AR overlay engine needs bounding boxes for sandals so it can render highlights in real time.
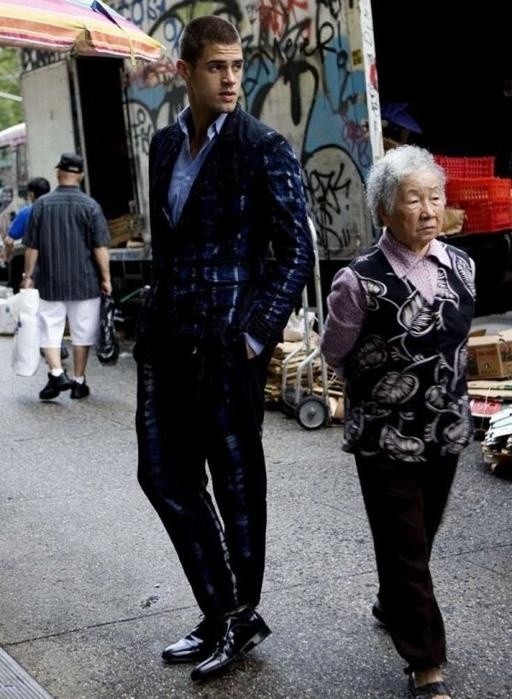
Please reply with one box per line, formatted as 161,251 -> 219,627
405,654 -> 454,699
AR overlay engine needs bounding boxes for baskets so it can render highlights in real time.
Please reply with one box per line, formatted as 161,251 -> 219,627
459,206 -> 512,232
444,178 -> 512,205
430,153 -> 495,178
381,100 -> 421,158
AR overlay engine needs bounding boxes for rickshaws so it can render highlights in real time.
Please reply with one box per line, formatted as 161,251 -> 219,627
278,216 -> 344,431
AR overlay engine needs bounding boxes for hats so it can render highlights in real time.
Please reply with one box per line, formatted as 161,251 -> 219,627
54,153 -> 86,174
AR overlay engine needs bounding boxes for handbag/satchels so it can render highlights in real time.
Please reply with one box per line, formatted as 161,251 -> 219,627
98,290 -> 119,366
13,284 -> 41,377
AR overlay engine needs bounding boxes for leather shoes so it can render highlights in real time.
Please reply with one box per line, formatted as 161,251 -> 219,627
157,612 -> 218,660
194,608 -> 270,683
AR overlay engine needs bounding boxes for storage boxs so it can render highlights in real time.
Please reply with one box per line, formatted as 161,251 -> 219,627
464,329 -> 512,378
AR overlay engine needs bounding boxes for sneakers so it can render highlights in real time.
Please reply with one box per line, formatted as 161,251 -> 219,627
70,373 -> 91,399
38,370 -> 70,400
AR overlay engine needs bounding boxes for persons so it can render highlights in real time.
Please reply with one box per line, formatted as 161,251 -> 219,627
3,153 -> 113,399
132,16 -> 316,681
321,144 -> 479,699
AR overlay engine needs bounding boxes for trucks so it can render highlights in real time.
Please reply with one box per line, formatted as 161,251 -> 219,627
0,0 -> 512,267
